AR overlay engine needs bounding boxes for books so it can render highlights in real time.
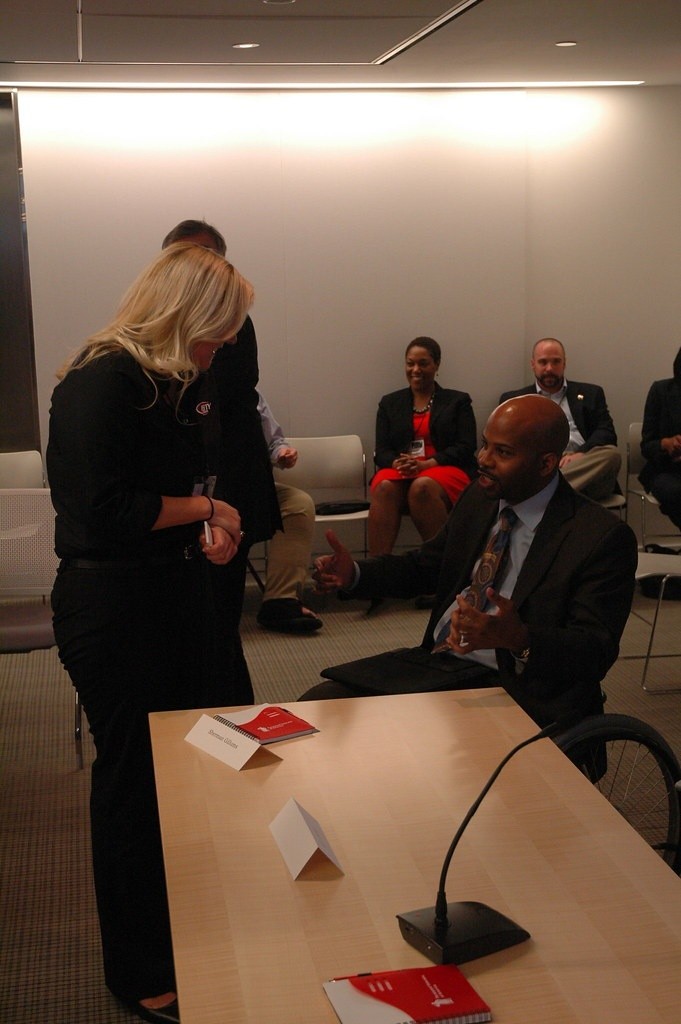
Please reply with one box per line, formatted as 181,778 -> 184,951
214,703 -> 315,744
322,964 -> 492,1024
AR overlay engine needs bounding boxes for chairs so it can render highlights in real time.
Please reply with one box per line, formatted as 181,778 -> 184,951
0,450 -> 84,770
634,552 -> 681,692
597,494 -> 626,520
626,423 -> 681,552
272,435 -> 370,558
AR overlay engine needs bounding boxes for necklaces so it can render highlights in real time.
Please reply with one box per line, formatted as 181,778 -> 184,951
412,392 -> 435,413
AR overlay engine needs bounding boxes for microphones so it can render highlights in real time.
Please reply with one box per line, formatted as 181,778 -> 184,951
394,722 -> 562,964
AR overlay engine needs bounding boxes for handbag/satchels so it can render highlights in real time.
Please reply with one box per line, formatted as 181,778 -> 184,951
320,646 -> 497,695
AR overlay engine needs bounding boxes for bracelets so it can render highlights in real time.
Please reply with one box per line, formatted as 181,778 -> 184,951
511,644 -> 530,662
203,494 -> 214,520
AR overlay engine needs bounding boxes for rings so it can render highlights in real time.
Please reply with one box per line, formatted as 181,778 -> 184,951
458,633 -> 464,644
240,531 -> 246,538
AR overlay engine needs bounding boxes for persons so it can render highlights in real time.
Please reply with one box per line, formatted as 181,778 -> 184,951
45,247 -> 245,1024
298,393 -> 637,785
499,338 -> 621,500
637,346 -> 681,530
368,337 -> 478,608
162,220 -> 323,632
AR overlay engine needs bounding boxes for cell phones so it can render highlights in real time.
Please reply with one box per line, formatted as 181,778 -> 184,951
204,521 -> 213,548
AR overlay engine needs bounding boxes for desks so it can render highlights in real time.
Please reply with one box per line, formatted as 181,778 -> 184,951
148,687 -> 681,1023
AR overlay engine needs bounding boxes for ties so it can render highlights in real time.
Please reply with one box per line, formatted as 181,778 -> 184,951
430,506 -> 516,655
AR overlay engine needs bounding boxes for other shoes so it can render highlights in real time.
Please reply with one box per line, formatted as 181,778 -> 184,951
256,598 -> 323,629
132,996 -> 179,1024
